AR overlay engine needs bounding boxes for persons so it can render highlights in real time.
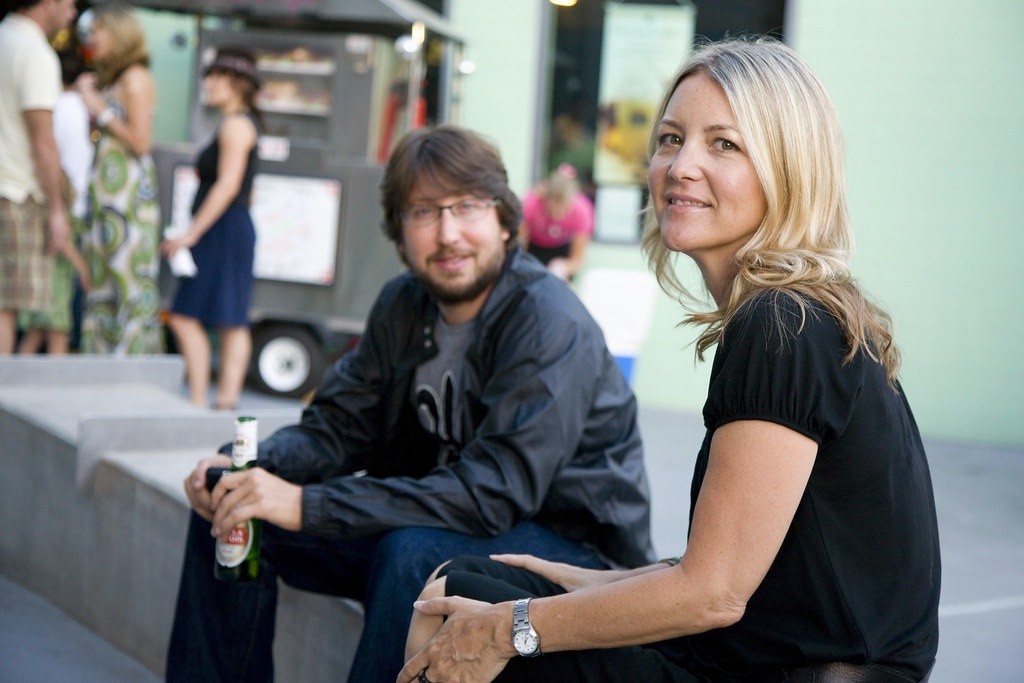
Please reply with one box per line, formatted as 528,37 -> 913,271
396,37 -> 941,683
14,52 -> 91,358
76,0 -> 162,357
0,0 -> 90,353
165,43 -> 263,410
165,123 -> 653,683
517,164 -> 593,285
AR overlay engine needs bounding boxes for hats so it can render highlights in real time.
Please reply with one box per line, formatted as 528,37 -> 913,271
203,46 -> 261,90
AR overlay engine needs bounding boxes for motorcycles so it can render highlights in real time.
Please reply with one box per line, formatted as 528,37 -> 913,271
73,0 -> 470,403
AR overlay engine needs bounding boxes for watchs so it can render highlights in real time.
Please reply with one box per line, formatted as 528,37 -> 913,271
509,596 -> 542,657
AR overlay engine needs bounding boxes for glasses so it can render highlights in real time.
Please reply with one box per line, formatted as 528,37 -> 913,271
400,198 -> 497,228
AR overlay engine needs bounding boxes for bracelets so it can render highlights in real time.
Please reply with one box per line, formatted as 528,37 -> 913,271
96,108 -> 116,127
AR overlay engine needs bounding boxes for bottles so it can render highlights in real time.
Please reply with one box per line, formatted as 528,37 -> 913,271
213,417 -> 261,583
